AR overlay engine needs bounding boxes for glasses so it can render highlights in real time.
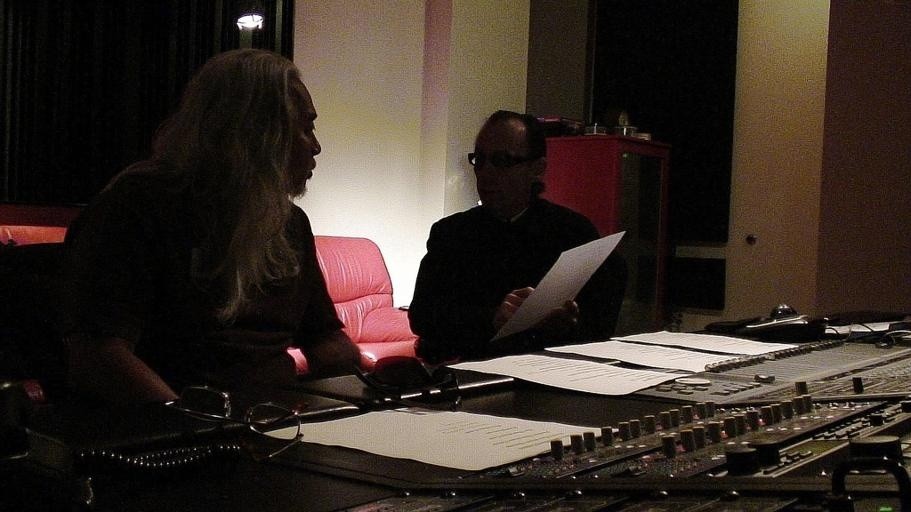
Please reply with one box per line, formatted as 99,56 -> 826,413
148,382 -> 309,465
466,148 -> 536,169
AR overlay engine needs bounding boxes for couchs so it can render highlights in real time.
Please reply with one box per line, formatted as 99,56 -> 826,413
1,226 -> 419,420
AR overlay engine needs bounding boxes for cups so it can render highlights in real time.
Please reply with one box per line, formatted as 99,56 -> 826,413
584,126 -> 608,136
611,126 -> 636,136
634,133 -> 652,141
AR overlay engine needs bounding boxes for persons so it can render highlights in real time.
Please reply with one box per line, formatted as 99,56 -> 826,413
27,48 -> 365,426
407,110 -> 629,367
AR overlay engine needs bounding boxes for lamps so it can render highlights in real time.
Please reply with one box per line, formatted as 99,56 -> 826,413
234,0 -> 265,33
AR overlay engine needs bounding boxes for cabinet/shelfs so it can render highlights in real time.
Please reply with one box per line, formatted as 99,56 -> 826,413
537,133 -> 671,338
